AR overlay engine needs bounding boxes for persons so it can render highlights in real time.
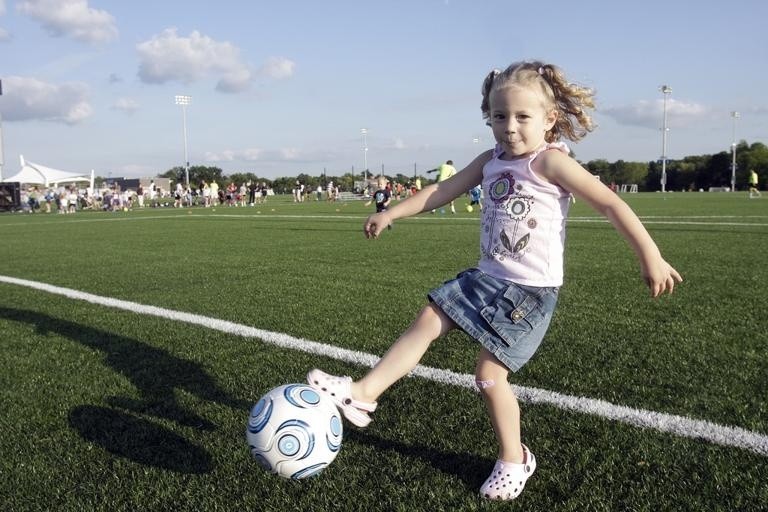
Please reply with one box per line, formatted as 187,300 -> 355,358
358,178 -> 421,201
317,184 -> 323,201
293,179 -> 313,202
466,186 -> 483,212
427,160 -> 457,214
305,60 -> 682,499
255,181 -> 274,205
29,183 -> 135,214
190,179 -> 255,207
364,176 -> 393,231
326,181 -> 339,202
748,169 -> 761,199
136,180 -> 190,208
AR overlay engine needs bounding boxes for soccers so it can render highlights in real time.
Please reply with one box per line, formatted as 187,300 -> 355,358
123,207 -> 129,212
466,205 -> 473,213
246,383 -> 343,481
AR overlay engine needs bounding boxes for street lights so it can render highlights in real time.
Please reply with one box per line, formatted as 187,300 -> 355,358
472,136 -> 480,157
729,109 -> 742,193
174,93 -> 192,185
359,126 -> 369,182
656,84 -> 673,192
250,167 -> 256,180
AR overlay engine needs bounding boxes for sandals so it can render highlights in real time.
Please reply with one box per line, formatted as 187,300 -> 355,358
306,369 -> 377,428
476,444 -> 536,502
